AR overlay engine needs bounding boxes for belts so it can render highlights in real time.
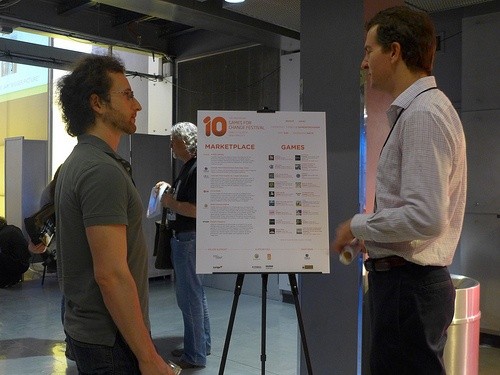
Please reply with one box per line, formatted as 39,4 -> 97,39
364,256 -> 408,272
171,228 -> 195,235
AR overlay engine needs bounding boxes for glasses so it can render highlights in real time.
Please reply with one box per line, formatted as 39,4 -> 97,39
102,91 -> 134,101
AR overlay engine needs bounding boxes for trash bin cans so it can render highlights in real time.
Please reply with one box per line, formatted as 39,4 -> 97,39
441,272 -> 479,373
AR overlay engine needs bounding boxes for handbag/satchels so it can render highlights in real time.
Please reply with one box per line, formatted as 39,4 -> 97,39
153,219 -> 174,269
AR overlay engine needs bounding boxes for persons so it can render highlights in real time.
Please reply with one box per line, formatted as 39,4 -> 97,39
0,218 -> 30,288
53,55 -> 171,375
155,122 -> 212,370
332,5 -> 466,375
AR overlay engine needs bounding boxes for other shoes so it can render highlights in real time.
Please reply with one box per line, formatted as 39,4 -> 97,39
171,358 -> 202,369
169,348 -> 211,357
